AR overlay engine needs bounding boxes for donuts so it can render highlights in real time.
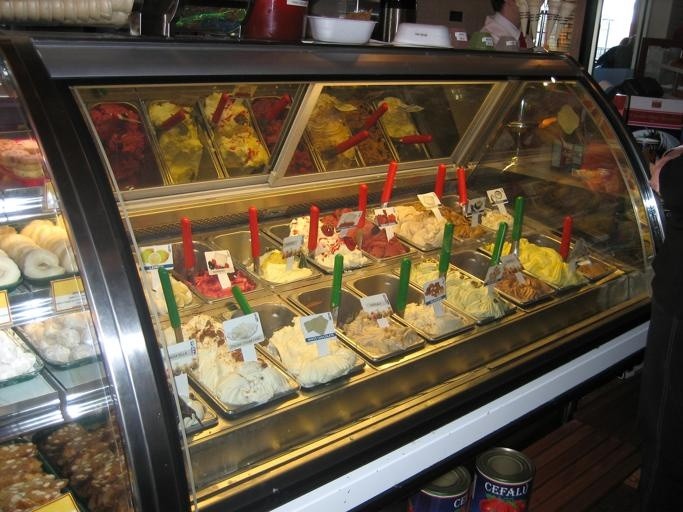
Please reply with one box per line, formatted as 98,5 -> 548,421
0,215 -> 79,287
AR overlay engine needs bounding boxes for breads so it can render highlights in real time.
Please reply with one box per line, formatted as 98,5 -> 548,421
0,442 -> 69,512
42,409 -> 135,512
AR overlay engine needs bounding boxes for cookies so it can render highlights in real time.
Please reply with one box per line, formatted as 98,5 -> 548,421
0,137 -> 45,187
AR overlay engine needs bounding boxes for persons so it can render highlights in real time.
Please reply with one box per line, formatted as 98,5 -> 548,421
635,144 -> 683,512
480,0 -> 522,44
597,38 -> 632,68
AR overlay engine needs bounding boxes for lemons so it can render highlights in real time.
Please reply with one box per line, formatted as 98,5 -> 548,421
142,248 -> 170,265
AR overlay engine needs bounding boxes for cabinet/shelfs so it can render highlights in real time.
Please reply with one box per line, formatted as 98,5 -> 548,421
1,32 -> 668,512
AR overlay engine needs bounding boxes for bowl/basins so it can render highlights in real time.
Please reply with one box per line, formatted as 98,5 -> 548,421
307,15 -> 379,46
393,22 -> 456,48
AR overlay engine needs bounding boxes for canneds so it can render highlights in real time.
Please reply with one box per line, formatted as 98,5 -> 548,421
406,464 -> 471,512
467,447 -> 535,511
